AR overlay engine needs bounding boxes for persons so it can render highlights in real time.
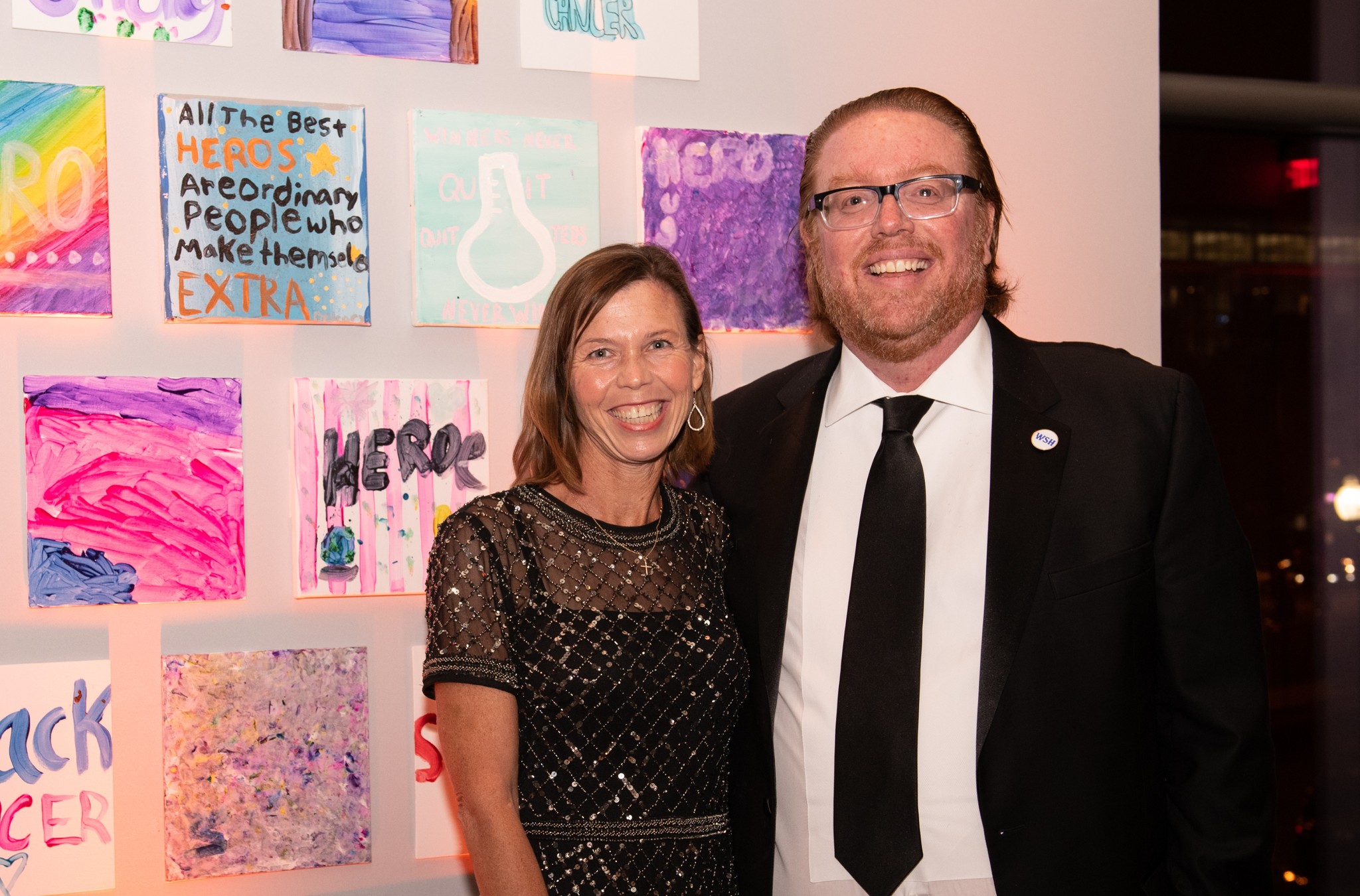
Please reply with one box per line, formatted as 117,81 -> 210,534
415,241 -> 757,896
697,85 -> 1269,896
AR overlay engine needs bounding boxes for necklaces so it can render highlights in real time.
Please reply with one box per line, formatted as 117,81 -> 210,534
566,487 -> 668,577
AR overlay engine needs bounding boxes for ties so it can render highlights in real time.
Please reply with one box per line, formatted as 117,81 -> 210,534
832,396 -> 940,896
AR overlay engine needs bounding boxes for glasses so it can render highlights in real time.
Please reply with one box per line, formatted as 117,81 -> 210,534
806,172 -> 992,230
